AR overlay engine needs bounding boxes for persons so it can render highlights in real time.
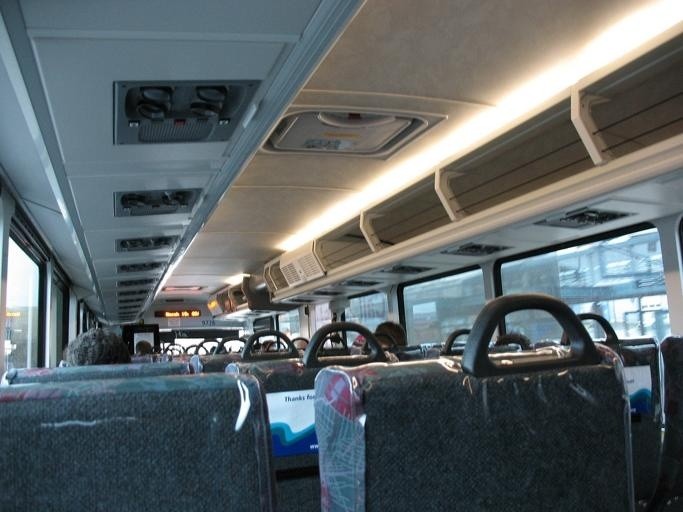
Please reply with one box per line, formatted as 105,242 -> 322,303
60,327 -> 131,366
261,321 -> 407,363
134,341 -> 151,358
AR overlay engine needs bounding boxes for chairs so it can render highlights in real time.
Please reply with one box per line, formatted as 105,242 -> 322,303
0,294 -> 681,512
